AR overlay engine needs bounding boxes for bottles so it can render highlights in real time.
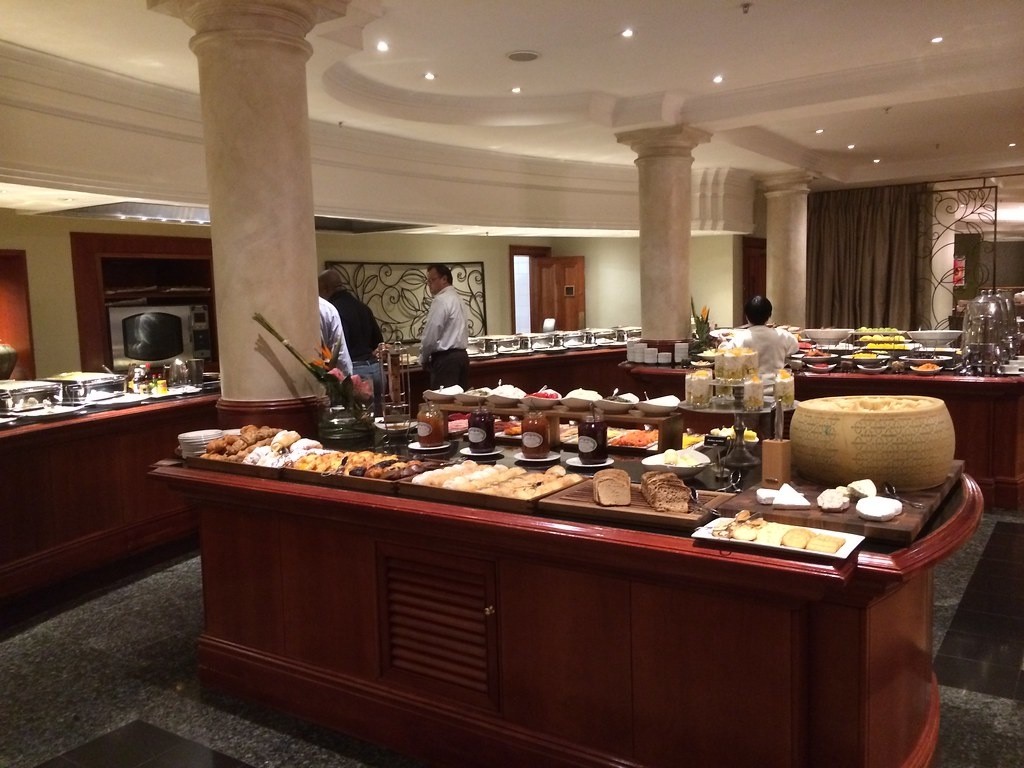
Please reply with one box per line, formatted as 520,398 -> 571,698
468,409 -> 495,453
521,411 -> 550,459
417,403 -> 445,448
127,358 -> 189,394
577,415 -> 607,465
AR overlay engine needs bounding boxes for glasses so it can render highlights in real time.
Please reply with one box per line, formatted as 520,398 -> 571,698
425,274 -> 442,284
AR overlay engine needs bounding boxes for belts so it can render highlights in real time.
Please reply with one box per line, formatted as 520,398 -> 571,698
352,358 -> 378,367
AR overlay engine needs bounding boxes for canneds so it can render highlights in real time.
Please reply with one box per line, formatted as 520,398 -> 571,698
467,408 -> 495,453
521,411 -> 550,459
417,404 -> 444,448
578,415 -> 608,465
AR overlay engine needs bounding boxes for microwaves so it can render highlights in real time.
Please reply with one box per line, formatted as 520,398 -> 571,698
108,305 -> 212,373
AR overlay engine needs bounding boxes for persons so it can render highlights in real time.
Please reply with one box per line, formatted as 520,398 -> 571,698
318,296 -> 353,376
717,296 -> 799,396
318,270 -> 384,417
419,263 -> 470,390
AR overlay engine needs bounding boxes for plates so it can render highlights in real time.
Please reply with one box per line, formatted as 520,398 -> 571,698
408,441 -> 451,450
566,456 -> 614,467
998,371 -> 1024,375
177,428 -> 241,460
383,339 -> 626,366
691,517 -> 866,559
0,392 -> 151,425
1019,367 -> 1024,369
513,451 -> 561,461
460,446 -> 505,455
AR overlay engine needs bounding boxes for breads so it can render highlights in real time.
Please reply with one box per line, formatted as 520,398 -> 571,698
756,479 -> 902,521
592,468 -> 632,506
711,512 -> 845,555
639,470 -> 691,513
200,425 -> 583,499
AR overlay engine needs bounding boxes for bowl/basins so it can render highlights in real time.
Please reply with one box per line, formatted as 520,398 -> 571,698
483,384 -> 526,407
203,372 -> 219,382
593,393 -> 639,413
519,389 -> 562,409
559,389 -> 603,409
641,448 -> 711,479
422,385 -> 464,404
1015,356 -> 1024,360
1009,360 -> 1024,367
454,387 -> 491,405
690,323 -> 963,375
1000,365 -> 1019,372
374,416 -> 418,433
745,437 -> 759,451
633,394 -> 680,415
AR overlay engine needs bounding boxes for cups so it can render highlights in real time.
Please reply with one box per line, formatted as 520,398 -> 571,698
627,338 -> 688,363
186,359 -> 204,390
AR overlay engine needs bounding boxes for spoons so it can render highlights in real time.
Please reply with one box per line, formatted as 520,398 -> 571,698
882,482 -> 925,509
688,486 -> 721,516
